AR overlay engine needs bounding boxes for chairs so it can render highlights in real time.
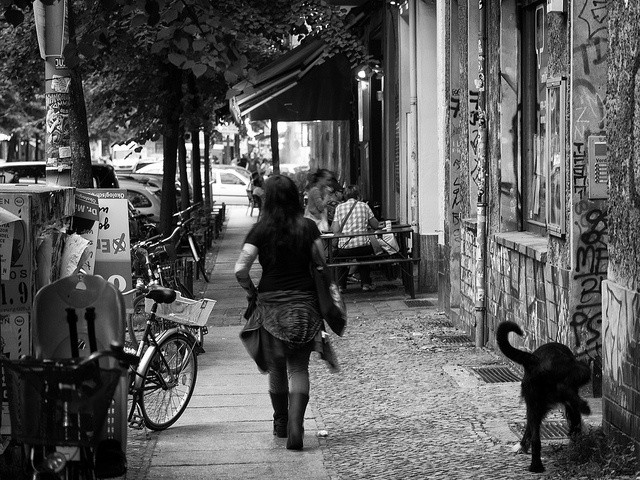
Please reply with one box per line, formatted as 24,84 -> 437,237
18,271 -> 128,458
251,195 -> 261,222
246,189 -> 253,216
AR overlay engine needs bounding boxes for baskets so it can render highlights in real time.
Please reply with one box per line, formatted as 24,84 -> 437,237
145,287 -> 216,328
141,257 -> 194,299
3,351 -> 121,448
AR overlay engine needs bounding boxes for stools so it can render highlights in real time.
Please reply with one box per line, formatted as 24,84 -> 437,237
210,201 -> 225,240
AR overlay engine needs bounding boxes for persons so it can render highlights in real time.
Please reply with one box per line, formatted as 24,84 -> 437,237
234,175 -> 327,449
305,169 -> 331,238
332,185 -> 378,292
246,171 -> 272,209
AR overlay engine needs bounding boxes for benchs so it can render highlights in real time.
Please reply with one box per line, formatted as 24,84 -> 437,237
321,228 -> 421,299
322,223 -> 411,292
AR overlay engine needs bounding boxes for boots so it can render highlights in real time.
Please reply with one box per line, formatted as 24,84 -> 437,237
286,392 -> 309,449
269,390 -> 290,438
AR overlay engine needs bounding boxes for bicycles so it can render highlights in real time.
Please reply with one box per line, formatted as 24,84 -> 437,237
127,232 -> 191,375
129,202 -> 210,283
78,268 -> 217,432
147,216 -> 210,357
1,273 -> 142,480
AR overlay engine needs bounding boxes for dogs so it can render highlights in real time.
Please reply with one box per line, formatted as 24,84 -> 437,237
495,320 -> 591,474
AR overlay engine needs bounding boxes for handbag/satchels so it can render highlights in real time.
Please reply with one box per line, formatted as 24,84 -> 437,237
313,266 -> 347,336
323,238 -> 339,258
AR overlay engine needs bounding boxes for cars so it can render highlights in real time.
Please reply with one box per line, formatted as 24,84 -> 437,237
0,159 -> 119,189
116,171 -> 164,199
112,172 -> 162,223
210,164 -> 254,206
